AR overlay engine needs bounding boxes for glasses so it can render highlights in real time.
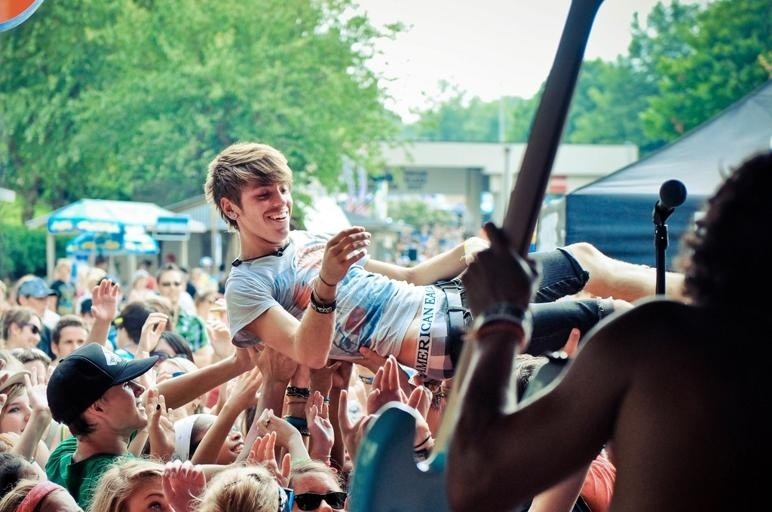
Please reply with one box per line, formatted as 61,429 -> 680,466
25,324 -> 40,335
150,352 -> 168,361
294,492 -> 347,510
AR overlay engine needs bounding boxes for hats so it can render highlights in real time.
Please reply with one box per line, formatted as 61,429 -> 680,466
0,348 -> 31,393
174,414 -> 201,461
17,277 -> 60,299
47,342 -> 159,426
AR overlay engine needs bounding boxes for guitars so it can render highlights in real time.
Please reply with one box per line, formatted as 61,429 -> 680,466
347,1 -> 602,511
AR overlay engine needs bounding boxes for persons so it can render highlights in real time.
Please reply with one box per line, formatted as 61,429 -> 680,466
205,141 -> 684,382
1,235 -> 617,510
445,149 -> 771,512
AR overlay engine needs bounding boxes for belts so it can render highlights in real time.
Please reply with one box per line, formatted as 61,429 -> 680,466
436,280 -> 465,378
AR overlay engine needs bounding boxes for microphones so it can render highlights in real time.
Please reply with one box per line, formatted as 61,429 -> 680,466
651,179 -> 688,224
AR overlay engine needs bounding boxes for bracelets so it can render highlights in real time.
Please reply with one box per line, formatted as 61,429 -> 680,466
471,301 -> 530,349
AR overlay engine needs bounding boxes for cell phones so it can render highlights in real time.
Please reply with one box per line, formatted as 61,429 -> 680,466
284,488 -> 294,512
